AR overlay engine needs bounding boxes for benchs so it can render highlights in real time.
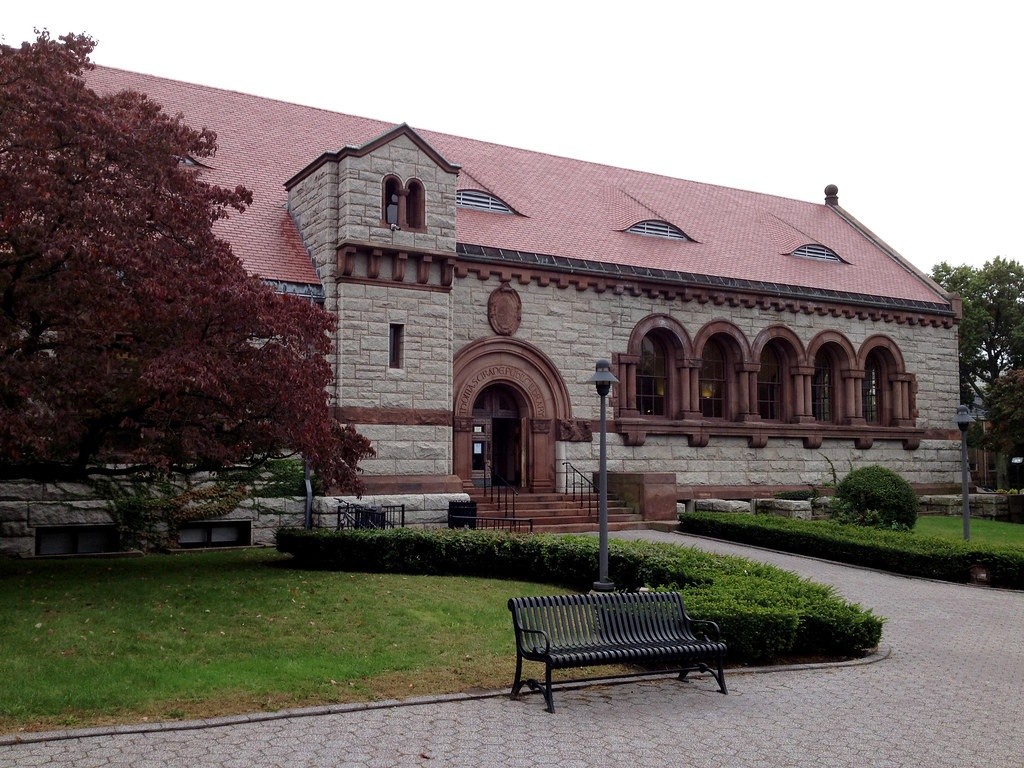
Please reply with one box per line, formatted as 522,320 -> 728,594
508,591 -> 728,715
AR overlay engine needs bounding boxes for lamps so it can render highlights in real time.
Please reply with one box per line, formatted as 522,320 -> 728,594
390,223 -> 401,230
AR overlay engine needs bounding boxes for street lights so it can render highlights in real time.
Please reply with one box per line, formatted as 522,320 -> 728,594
953,405 -> 976,544
589,358 -> 621,597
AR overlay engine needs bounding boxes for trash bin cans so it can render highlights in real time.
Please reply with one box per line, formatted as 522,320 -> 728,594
448,499 -> 477,529
